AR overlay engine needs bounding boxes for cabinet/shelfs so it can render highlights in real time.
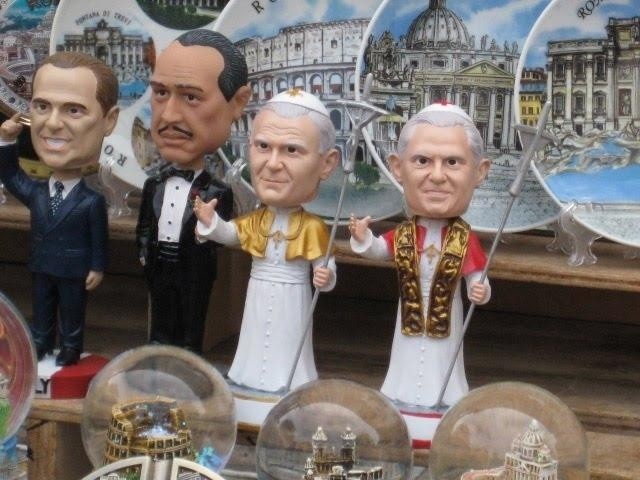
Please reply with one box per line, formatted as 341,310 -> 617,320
0,200 -> 640,479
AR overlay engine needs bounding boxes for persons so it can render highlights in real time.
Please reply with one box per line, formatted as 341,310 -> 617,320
134,30 -> 252,354
193,86 -> 339,393
348,102 -> 492,406
0,49 -> 121,367
